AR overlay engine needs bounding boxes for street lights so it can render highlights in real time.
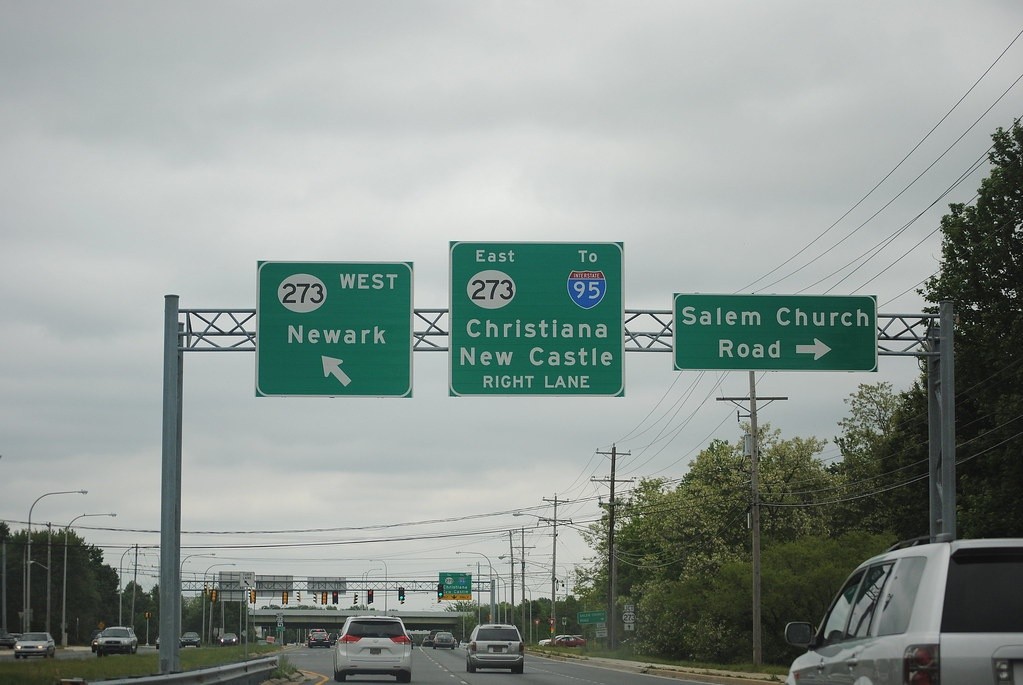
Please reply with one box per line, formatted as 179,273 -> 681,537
455,513 -> 556,635
26,490 -> 235,644
361,560 -> 387,616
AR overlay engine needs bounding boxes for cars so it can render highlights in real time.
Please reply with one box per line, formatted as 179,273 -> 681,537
333,615 -> 414,682
155,632 -> 201,649
422,630 -> 470,649
8,632 -> 55,659
308,628 -> 339,648
538,635 -> 587,647
220,633 -> 238,646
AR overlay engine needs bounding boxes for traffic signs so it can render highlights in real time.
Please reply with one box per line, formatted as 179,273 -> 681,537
448,241 -> 627,398
577,610 -> 608,624
256,260 -> 414,398
672,292 -> 878,371
439,572 -> 472,600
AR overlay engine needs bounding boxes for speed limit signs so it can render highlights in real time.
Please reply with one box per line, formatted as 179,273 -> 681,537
622,611 -> 637,624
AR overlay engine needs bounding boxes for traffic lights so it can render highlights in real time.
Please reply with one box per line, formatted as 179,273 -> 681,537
210,588 -> 374,605
398,587 -> 404,601
204,584 -> 209,595
438,584 -> 444,598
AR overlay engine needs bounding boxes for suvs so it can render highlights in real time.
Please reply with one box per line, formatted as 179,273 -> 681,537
91,626 -> 138,657
466,623 -> 524,673
784,533 -> 1023,685
